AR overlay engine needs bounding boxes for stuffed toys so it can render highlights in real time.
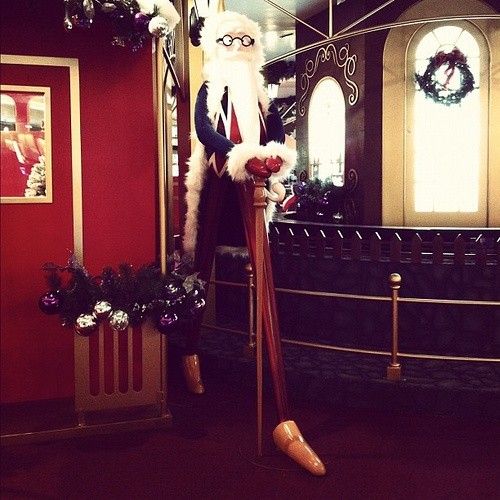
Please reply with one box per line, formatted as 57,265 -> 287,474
176,12 -> 326,475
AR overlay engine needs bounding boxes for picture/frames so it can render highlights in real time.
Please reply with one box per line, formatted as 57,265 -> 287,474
1,83 -> 54,204
